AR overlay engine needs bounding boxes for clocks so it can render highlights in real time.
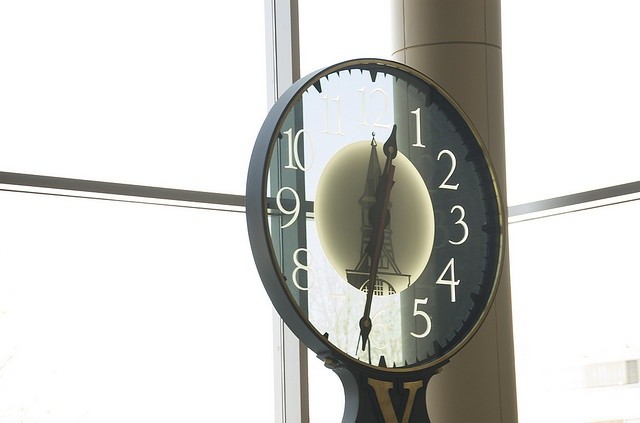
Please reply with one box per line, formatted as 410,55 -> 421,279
245,59 -> 505,423
